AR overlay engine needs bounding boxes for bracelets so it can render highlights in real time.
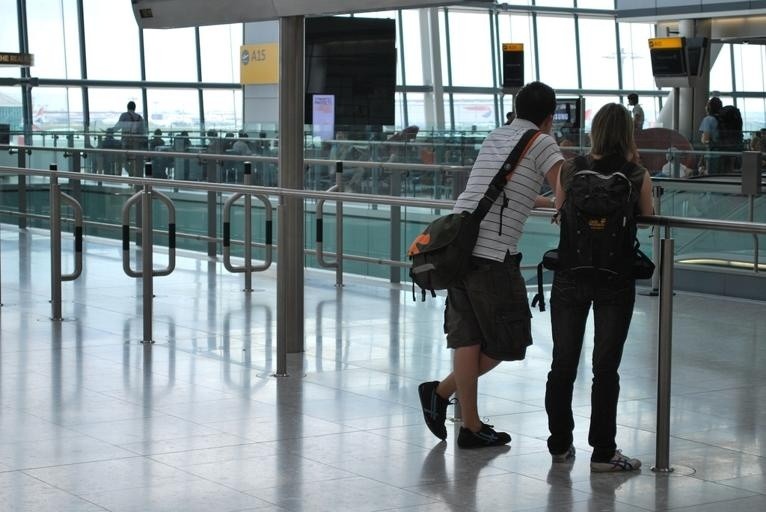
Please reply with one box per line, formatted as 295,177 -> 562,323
550,194 -> 556,207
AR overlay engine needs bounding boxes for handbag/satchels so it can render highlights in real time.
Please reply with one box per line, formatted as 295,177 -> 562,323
405,211 -> 483,293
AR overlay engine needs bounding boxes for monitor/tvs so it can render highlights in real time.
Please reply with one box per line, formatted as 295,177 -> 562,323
650,48 -> 687,77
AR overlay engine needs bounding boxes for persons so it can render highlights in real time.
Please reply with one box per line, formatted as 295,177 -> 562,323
415,82 -> 564,446
628,93 -> 646,127
545,455 -> 641,511
418,440 -> 512,511
545,104 -> 655,471
700,97 -> 727,176
756,128 -> 766,168
750,131 -> 761,152
505,111 -> 515,125
110,102 -> 145,176
662,144 -> 692,177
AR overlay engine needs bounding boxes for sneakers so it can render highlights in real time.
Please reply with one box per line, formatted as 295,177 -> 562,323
589,449 -> 642,475
458,423 -> 513,450
551,441 -> 578,464
417,379 -> 459,441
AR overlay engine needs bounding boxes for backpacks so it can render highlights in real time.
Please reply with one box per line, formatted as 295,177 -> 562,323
543,152 -> 656,290
707,104 -> 744,158
